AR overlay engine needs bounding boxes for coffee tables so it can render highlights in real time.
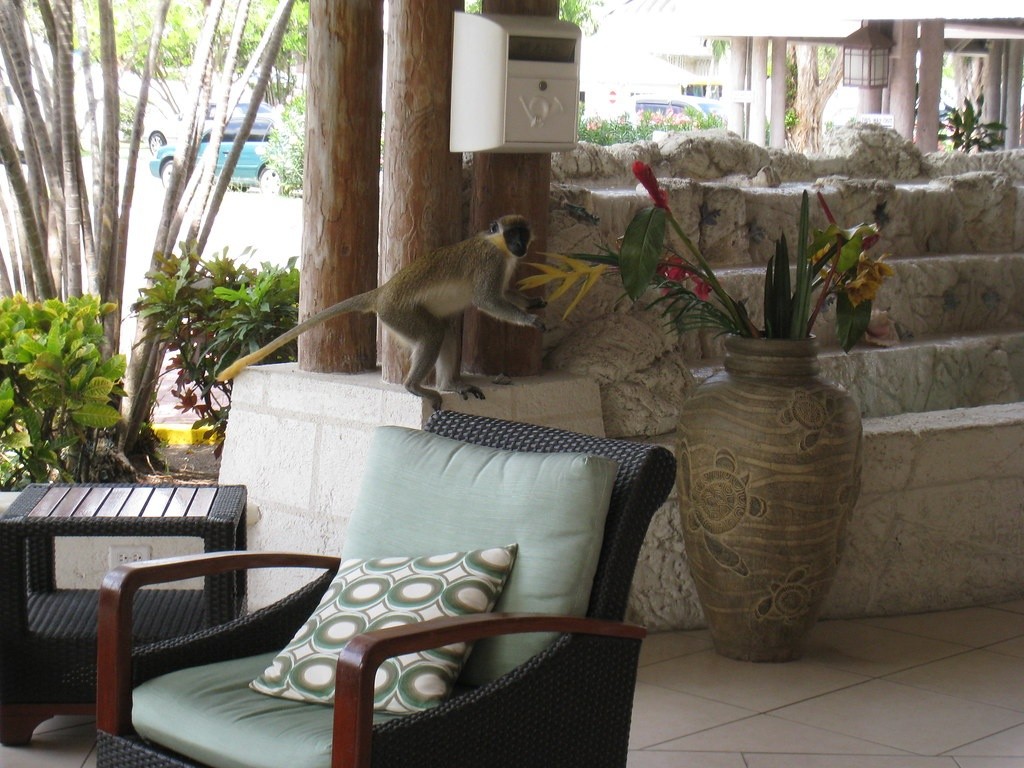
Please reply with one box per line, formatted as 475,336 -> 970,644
0,483 -> 246,747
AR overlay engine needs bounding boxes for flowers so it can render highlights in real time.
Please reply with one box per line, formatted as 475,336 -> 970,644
514,159 -> 893,353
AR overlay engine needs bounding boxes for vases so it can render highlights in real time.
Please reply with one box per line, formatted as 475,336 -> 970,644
673,332 -> 867,662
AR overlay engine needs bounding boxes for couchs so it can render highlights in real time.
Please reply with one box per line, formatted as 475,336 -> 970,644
96,408 -> 675,768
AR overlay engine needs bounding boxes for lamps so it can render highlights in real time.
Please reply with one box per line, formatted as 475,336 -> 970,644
842,19 -> 898,89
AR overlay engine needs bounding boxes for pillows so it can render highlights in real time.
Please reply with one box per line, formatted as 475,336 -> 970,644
249,544 -> 521,716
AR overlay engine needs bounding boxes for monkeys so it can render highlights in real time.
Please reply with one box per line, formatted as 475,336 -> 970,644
215,214 -> 548,410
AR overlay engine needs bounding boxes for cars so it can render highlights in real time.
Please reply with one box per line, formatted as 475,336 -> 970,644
148,114 -> 286,194
139,97 -> 280,159
596,95 -> 724,131
